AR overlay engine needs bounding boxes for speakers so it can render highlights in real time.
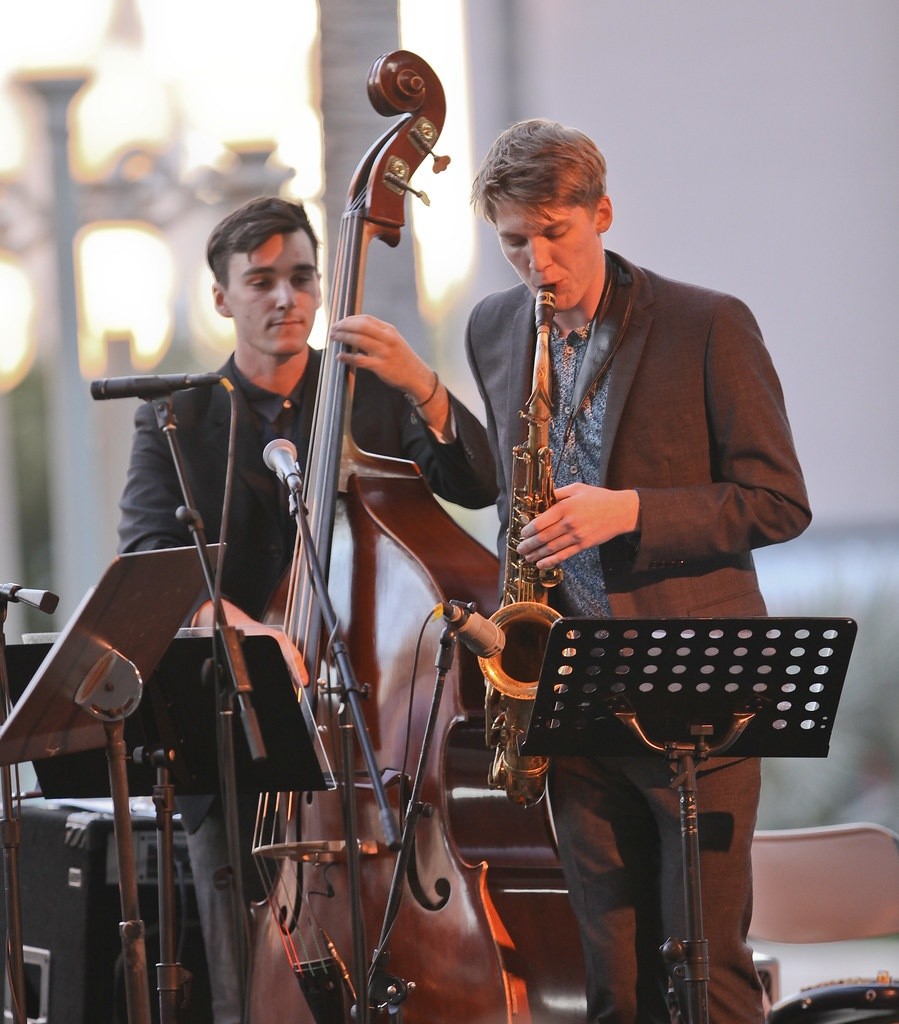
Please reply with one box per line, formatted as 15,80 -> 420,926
0,802 -> 213,1024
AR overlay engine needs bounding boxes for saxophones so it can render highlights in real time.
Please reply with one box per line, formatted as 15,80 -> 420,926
473,274 -> 575,809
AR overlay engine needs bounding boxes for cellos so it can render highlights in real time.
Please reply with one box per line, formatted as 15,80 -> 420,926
224,48 -> 534,1024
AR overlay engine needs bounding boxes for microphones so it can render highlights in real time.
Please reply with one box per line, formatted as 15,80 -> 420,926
442,603 -> 506,658
91,373 -> 226,402
262,439 -> 303,494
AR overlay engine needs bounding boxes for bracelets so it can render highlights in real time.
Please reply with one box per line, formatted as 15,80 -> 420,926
406,372 -> 438,425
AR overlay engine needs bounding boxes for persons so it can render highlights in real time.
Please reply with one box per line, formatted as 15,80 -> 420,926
464,117 -> 815,1024
117,194 -> 499,1024
